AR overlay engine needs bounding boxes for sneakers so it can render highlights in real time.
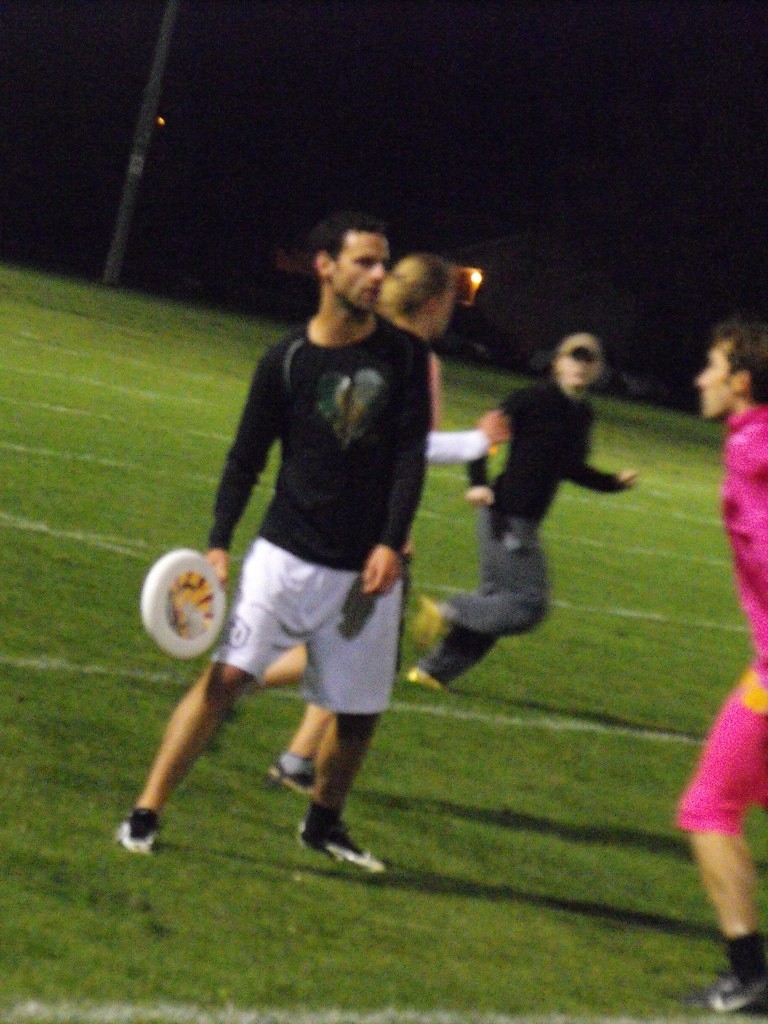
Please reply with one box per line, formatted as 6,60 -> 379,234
298,820 -> 386,874
115,819 -> 155,858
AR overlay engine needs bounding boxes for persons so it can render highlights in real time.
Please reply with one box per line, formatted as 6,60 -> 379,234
672,316 -> 768,1015
224,255 -> 638,799
118,209 -> 428,872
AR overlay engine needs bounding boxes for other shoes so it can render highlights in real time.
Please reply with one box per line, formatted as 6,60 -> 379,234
408,665 -> 450,693
410,594 -> 449,649
683,973 -> 768,1013
266,759 -> 319,794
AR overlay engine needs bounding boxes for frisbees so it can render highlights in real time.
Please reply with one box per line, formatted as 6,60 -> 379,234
142,550 -> 226,657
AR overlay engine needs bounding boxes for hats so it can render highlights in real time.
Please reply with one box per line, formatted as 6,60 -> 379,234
562,334 -> 603,362
